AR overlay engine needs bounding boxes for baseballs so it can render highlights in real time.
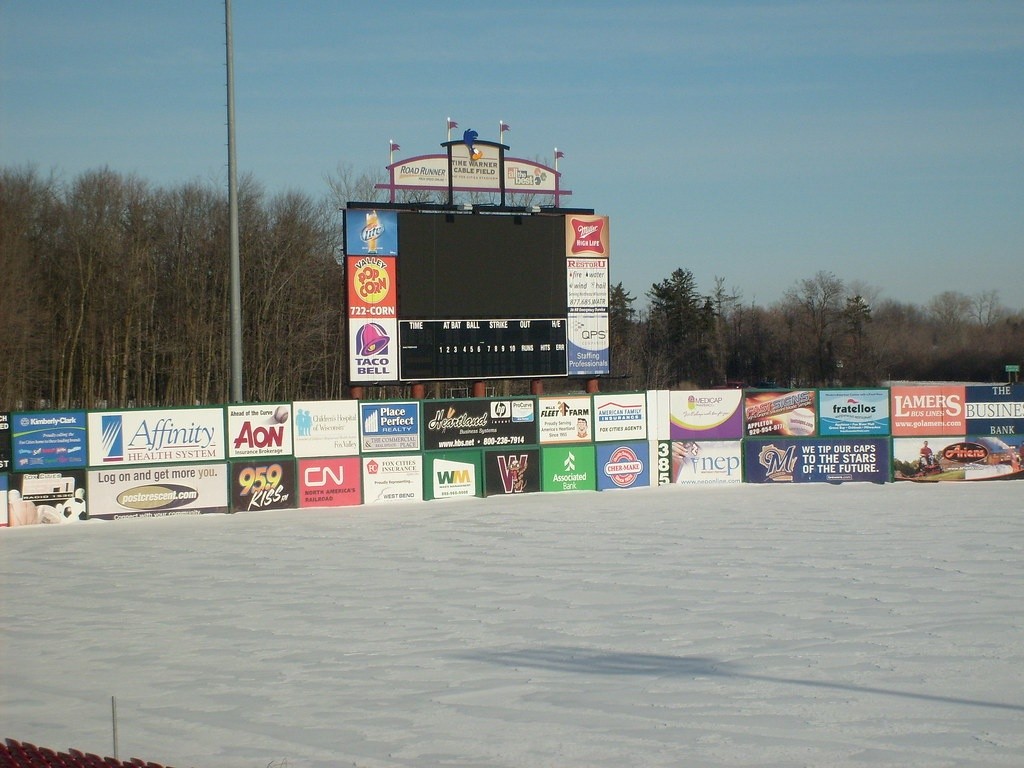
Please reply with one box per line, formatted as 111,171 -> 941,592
789,408 -> 815,435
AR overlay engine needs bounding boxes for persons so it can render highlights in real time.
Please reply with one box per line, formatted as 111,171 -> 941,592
920,440 -> 934,467
